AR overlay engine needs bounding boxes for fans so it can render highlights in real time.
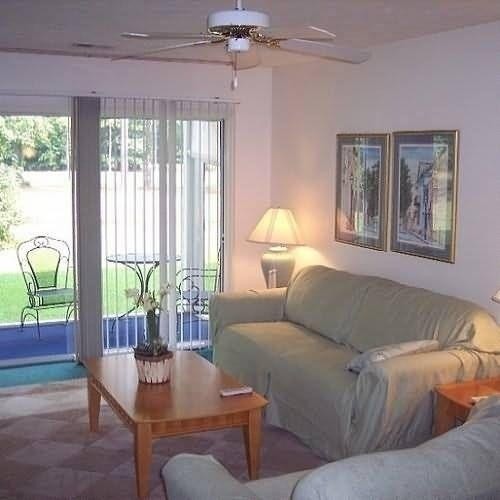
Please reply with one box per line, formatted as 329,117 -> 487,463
111,0 -> 371,64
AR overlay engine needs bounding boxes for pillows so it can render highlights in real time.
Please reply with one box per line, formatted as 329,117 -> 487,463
347,340 -> 439,373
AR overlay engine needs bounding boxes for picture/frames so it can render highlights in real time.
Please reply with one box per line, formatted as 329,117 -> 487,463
334,133 -> 389,252
389,129 -> 460,264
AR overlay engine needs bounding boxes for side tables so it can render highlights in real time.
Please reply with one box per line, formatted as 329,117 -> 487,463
432,376 -> 500,438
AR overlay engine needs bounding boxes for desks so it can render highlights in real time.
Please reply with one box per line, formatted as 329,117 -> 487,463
107,254 -> 182,333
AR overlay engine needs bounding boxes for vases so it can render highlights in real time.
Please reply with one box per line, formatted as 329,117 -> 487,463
135,350 -> 173,384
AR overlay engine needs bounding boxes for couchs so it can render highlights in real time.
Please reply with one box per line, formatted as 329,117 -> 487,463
210,264 -> 500,461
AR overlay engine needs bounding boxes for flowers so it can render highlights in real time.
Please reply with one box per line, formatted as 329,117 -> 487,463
124,283 -> 172,356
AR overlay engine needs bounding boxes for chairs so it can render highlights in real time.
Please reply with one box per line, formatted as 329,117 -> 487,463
17,236 -> 79,340
176,234 -> 224,332
161,394 -> 500,500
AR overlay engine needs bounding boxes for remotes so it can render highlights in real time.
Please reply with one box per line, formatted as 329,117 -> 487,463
220,386 -> 253,396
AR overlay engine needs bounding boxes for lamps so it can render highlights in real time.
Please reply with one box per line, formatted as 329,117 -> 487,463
246,206 -> 305,288
225,38 -> 251,88
